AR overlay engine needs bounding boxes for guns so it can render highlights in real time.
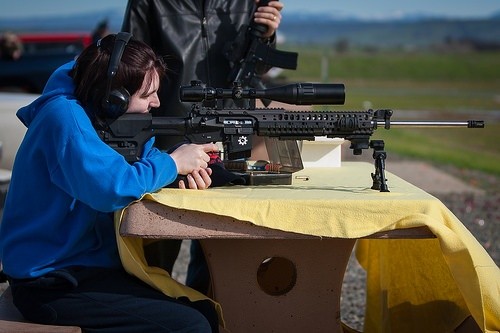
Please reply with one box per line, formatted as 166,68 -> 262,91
215,0 -> 299,107
95,77 -> 485,192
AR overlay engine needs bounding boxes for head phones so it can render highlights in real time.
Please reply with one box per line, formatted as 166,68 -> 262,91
101,32 -> 134,117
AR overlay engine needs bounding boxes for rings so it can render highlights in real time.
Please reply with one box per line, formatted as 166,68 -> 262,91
273,16 -> 276,21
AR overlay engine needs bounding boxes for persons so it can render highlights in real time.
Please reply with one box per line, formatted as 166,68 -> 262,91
122,0 -> 284,292
0,32 -> 219,333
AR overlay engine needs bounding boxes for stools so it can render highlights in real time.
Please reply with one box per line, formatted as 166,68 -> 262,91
0,281 -> 84,333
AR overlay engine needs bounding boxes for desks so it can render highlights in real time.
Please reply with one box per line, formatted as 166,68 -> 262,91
113,160 -> 500,333
